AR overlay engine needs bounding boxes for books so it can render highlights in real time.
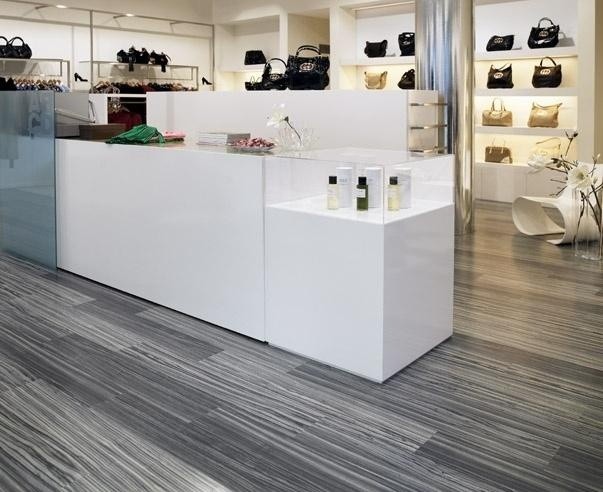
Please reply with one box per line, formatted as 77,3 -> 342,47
195,132 -> 251,150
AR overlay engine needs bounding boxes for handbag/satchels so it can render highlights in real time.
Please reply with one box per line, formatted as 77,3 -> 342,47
527,100 -> 564,129
398,31 -> 417,56
149,50 -> 170,66
261,57 -> 288,91
244,76 -> 263,91
486,34 -> 515,52
527,137 -> 563,167
484,135 -> 513,165
480,98 -> 513,128
244,49 -> 267,66
8,36 -> 33,60
362,70 -> 388,89
531,55 -> 563,89
0,35 -> 16,59
485,62 -> 515,89
245,75 -> 257,91
364,39 -> 388,58
116,49 -> 136,64
128,45 -> 151,64
398,68 -> 417,90
527,17 -> 560,50
285,43 -> 330,91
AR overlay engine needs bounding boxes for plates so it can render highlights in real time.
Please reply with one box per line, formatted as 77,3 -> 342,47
225,142 -> 281,152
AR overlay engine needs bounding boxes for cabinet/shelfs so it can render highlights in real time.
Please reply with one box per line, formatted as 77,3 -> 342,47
472,0 -> 595,205
0,1 -> 215,93
219,14 -> 287,90
332,0 -> 415,90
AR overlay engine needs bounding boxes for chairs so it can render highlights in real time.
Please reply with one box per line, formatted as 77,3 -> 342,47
506,161 -> 603,245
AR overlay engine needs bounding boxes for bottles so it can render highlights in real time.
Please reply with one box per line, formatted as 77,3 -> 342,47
328,176 -> 338,209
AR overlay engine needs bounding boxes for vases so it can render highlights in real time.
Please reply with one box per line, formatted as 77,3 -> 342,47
575,194 -> 602,261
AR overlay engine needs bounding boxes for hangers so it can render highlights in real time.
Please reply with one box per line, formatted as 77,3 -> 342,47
89,80 -> 193,93
11,75 -> 71,91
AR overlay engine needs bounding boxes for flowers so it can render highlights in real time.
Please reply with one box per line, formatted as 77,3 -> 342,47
525,129 -> 602,237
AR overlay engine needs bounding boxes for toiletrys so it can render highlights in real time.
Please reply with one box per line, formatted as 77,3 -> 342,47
326,176 -> 340,210
329,176 -> 339,210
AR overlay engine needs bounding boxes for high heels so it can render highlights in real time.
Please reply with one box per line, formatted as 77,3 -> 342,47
74,72 -> 88,82
202,76 -> 213,86
